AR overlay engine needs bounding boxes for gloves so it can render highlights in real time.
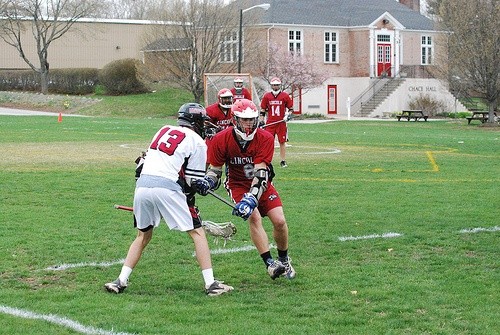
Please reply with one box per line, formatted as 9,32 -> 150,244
283,113 -> 292,122
191,176 -> 215,197
259,121 -> 266,130
232,193 -> 259,221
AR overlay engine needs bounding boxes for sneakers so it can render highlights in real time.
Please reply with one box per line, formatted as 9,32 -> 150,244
279,256 -> 295,279
104,277 -> 128,294
266,259 -> 285,280
205,281 -> 235,297
280,160 -> 288,168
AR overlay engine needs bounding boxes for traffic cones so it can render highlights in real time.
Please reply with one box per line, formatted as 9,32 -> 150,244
57,113 -> 62,122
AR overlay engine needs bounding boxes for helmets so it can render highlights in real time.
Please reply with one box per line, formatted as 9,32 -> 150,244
231,99 -> 259,141
233,77 -> 244,90
217,89 -> 234,109
177,103 -> 211,132
270,78 -> 282,96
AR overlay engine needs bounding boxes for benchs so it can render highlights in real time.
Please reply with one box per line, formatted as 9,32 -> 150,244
466,117 -> 500,124
396,116 -> 428,121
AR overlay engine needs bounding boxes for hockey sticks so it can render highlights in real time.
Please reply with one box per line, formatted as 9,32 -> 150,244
259,115 -> 301,128
114,204 -> 236,239
205,188 -> 240,214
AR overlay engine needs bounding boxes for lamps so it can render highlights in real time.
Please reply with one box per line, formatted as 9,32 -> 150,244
383,20 -> 389,24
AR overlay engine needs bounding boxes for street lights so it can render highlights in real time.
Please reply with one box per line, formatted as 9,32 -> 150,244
238,3 -> 270,73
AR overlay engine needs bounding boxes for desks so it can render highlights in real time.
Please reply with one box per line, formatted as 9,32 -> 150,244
401,110 -> 424,121
472,112 -> 497,118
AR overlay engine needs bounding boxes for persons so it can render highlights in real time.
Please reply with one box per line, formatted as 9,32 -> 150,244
190,98 -> 296,281
256,78 -> 295,167
104,103 -> 235,296
230,77 -> 252,104
205,88 -> 237,196
